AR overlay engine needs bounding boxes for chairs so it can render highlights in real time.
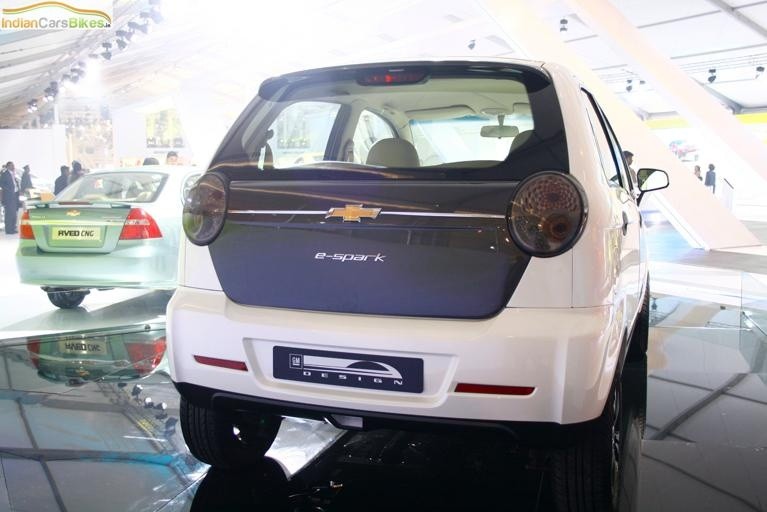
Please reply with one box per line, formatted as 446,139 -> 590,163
509,129 -> 534,152
366,138 -> 420,168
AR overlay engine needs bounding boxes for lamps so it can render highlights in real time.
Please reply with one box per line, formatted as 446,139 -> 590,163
26,0 -> 163,114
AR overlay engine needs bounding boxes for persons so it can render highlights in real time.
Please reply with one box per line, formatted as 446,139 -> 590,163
692,164 -> 702,181
52,164 -> 69,195
163,151 -> 178,165
20,164 -> 32,194
620,149 -> 638,185
0,161 -> 20,236
705,161 -> 716,195
65,160 -> 84,187
124,157 -> 161,201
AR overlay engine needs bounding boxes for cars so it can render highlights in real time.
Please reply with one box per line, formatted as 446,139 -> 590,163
165,57 -> 670,506
14,164 -> 208,310
